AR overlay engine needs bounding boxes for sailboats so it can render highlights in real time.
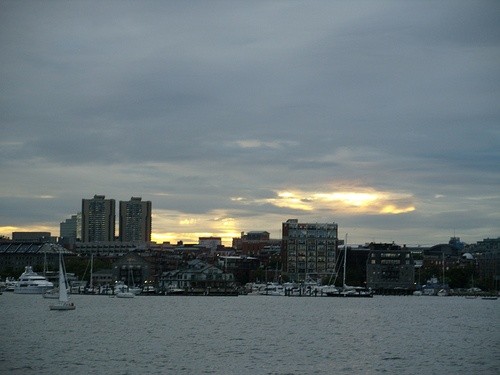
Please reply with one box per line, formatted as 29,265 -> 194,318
116,262 -> 140,298
327,233 -> 373,297
48,250 -> 75,311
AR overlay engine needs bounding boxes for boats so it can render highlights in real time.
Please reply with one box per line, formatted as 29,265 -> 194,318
245,282 -> 338,297
14,266 -> 54,294
